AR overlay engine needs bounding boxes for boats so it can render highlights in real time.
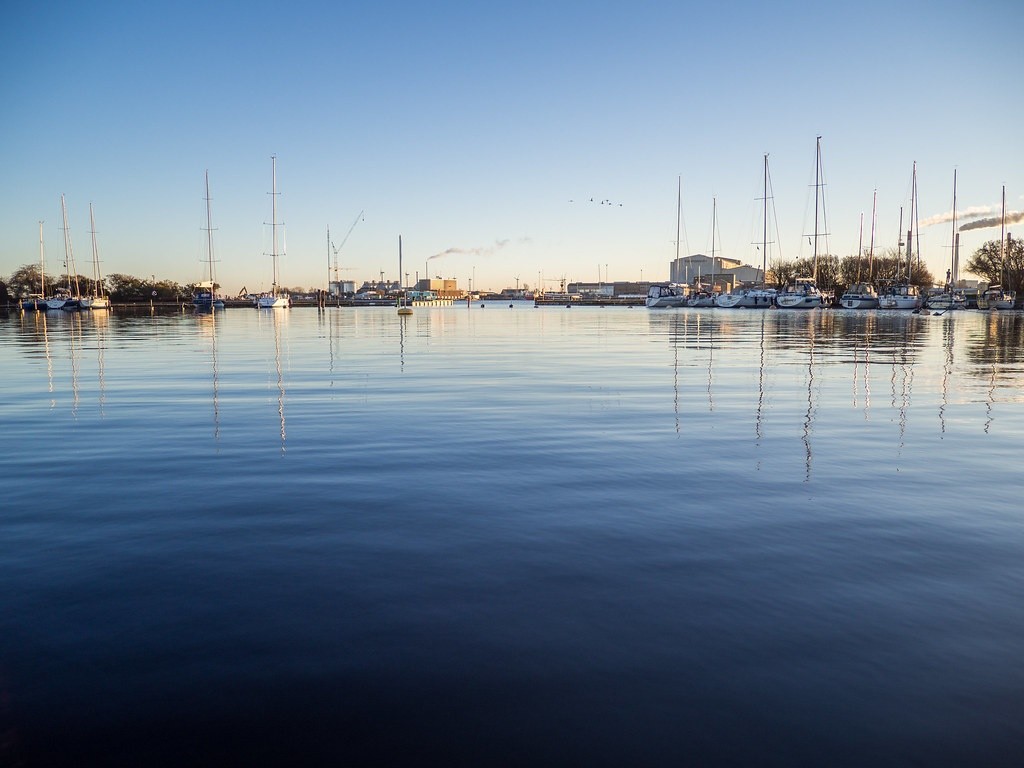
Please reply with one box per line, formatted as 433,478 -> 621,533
76,298 -> 94,308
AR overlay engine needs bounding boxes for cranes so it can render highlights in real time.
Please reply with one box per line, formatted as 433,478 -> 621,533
543,278 -> 566,292
331,209 -> 365,298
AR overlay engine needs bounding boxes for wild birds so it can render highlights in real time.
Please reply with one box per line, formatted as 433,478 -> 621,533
568,197 -> 623,209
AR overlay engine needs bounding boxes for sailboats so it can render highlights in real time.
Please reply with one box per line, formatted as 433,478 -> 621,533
716,152 -> 774,307
644,176 -> 689,308
77,202 -> 111,308
775,135 -> 833,308
60,193 -> 81,305
18,218 -> 65,310
686,198 -> 719,308
191,169 -> 225,312
926,169 -> 970,309
839,188 -> 879,308
256,153 -> 290,308
976,186 -> 1016,310
877,161 -> 923,310
